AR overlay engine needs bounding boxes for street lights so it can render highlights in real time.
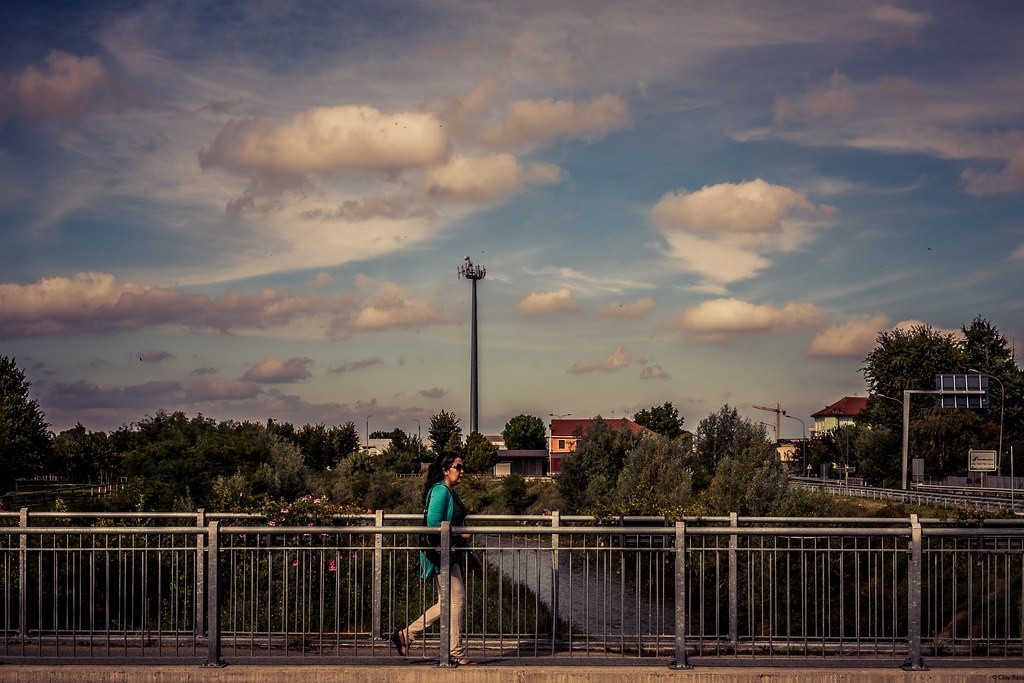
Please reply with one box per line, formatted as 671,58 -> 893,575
413,417 -> 421,461
878,392 -> 905,491
549,412 -> 571,420
824,405 -> 848,490
786,415 -> 806,478
759,422 -> 777,475
366,413 -> 372,465
956,369 -> 1010,491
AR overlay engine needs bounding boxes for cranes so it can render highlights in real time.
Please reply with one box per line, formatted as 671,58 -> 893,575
751,398 -> 783,442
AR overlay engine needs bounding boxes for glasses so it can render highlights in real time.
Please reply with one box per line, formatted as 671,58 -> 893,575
451,463 -> 466,472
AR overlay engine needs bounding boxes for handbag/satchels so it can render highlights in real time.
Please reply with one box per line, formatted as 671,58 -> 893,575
418,484 -> 468,570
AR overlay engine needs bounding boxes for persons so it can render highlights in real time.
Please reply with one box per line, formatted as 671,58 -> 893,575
392,451 -> 479,667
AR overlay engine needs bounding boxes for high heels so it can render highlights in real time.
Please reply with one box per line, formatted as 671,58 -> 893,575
449,656 -> 475,667
391,629 -> 412,662
